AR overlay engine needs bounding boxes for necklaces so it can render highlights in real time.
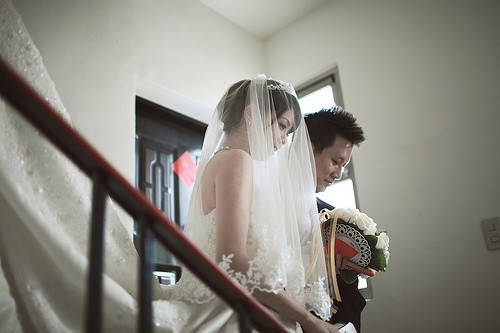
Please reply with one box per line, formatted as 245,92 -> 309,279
211,147 -> 230,153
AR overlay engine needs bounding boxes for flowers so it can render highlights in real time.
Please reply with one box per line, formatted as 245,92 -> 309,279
317,206 -> 390,285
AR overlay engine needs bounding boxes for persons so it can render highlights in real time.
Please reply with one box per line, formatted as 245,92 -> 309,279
292,106 -> 368,333
186,74 -> 343,333
